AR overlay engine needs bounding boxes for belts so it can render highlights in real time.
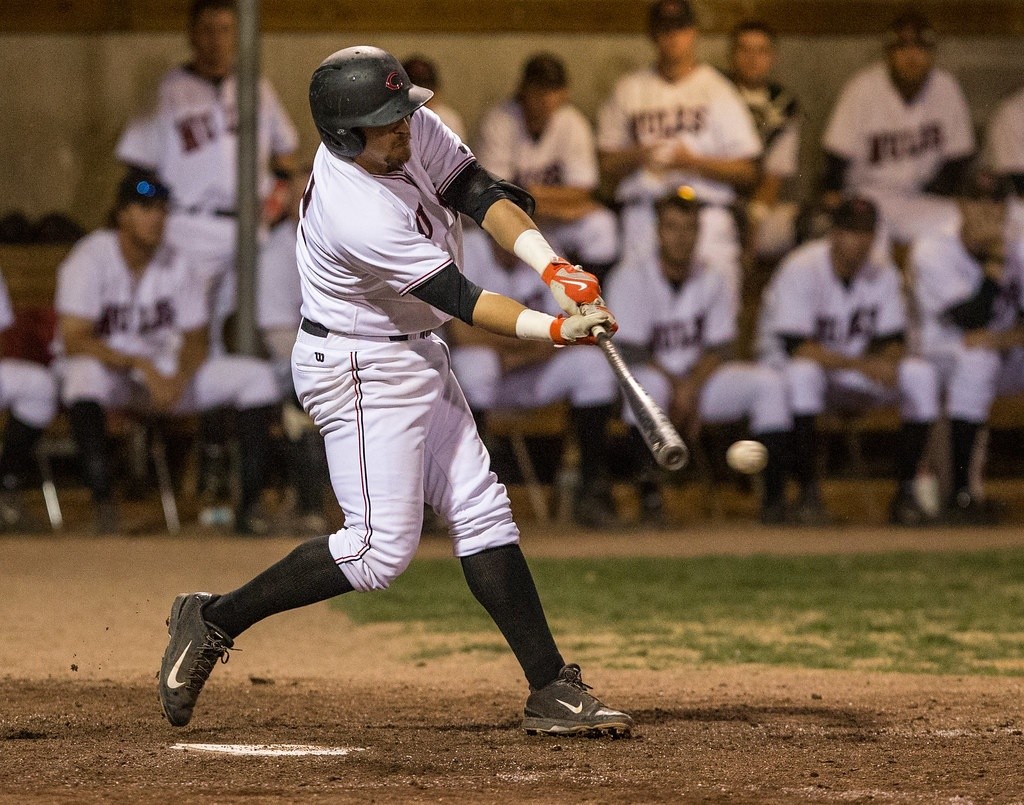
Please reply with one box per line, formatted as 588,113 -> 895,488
299,318 -> 434,341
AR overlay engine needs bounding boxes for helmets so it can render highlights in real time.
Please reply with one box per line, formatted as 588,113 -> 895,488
309,46 -> 435,158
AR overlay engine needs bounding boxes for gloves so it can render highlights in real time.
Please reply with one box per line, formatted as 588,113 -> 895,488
549,305 -> 618,348
541,257 -> 605,317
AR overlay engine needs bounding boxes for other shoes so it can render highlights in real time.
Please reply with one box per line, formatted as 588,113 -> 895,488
96,500 -> 117,532
761,488 -> 785,523
941,494 -> 997,525
295,509 -> 327,536
793,485 -> 822,524
235,505 -> 269,536
889,495 -> 930,525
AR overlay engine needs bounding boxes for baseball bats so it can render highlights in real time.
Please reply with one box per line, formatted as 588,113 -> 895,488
579,302 -> 690,473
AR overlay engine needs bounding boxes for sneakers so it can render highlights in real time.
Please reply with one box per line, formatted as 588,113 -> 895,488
155,592 -> 241,727
524,664 -> 633,736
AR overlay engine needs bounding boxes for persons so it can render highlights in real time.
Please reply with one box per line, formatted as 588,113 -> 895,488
46,169 -> 280,535
598,185 -> 790,533
256,167 -> 341,534
0,271 -> 60,533
903,181 -> 1024,523
594,0 -> 764,255
155,45 -> 636,740
477,52 -> 620,287
397,54 -> 468,146
427,181 -> 621,530
720,21 -> 805,271
752,200 -> 939,523
821,15 -> 977,246
112,0 -> 300,291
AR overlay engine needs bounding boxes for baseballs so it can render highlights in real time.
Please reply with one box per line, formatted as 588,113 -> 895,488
725,440 -> 769,475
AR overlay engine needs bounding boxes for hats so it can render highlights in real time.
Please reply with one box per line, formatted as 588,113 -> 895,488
881,16 -> 936,49
125,183 -> 167,207
656,189 -> 704,212
647,0 -> 692,37
834,200 -> 876,231
526,50 -> 566,86
961,169 -> 1006,202
